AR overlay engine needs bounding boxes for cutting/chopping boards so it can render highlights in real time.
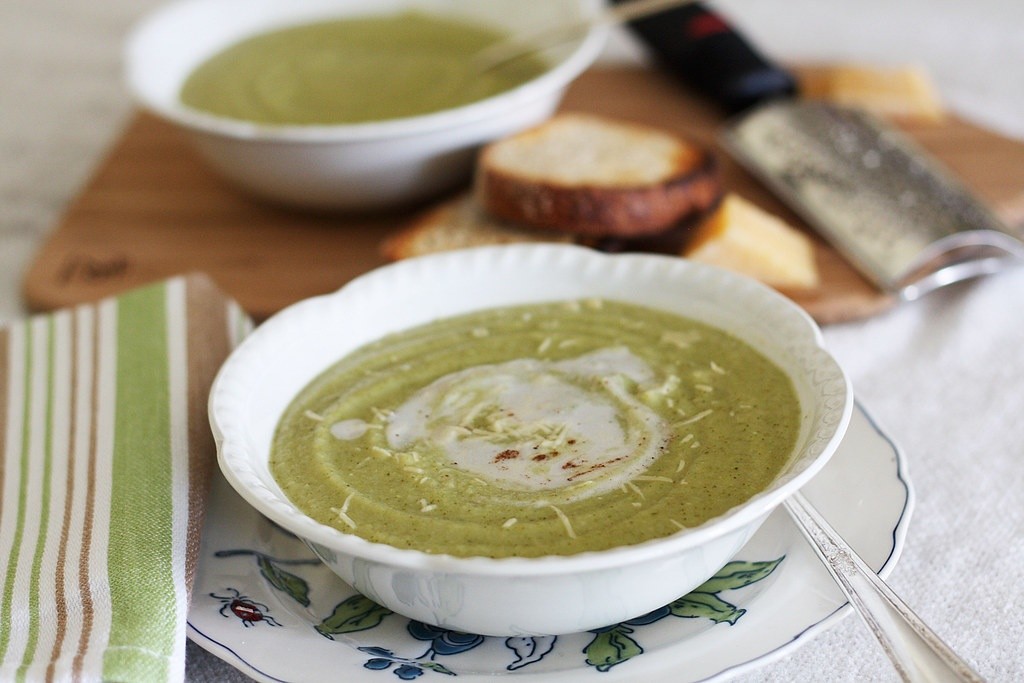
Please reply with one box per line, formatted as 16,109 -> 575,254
24,62 -> 1024,322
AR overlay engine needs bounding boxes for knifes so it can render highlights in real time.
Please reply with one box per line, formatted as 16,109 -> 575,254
606,1 -> 1023,300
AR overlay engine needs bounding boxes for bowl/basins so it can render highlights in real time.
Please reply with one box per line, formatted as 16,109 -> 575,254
123,0 -> 607,208
209,243 -> 852,635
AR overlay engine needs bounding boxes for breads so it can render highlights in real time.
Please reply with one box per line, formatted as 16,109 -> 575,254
380,108 -> 821,292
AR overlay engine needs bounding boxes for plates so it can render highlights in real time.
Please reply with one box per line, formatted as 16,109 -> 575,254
185,400 -> 910,683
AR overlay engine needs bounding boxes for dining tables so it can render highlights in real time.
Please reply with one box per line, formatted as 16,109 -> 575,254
21,58 -> 1024,683
